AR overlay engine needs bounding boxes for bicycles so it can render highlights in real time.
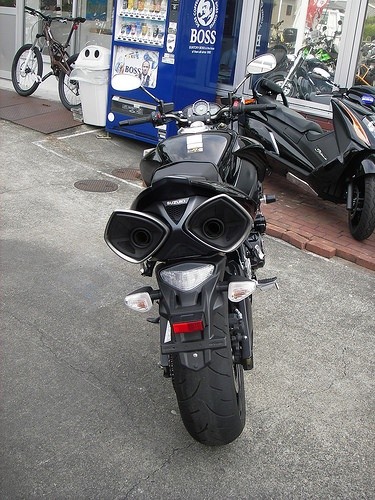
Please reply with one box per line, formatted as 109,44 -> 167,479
12,4 -> 88,113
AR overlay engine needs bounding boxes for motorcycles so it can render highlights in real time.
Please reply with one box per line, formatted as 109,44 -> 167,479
258,19 -> 374,106
109,53 -> 279,450
237,65 -> 375,243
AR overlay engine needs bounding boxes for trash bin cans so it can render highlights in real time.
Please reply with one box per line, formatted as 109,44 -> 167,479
69,45 -> 112,126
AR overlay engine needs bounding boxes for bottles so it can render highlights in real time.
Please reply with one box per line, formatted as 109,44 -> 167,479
119,0 -> 166,39
